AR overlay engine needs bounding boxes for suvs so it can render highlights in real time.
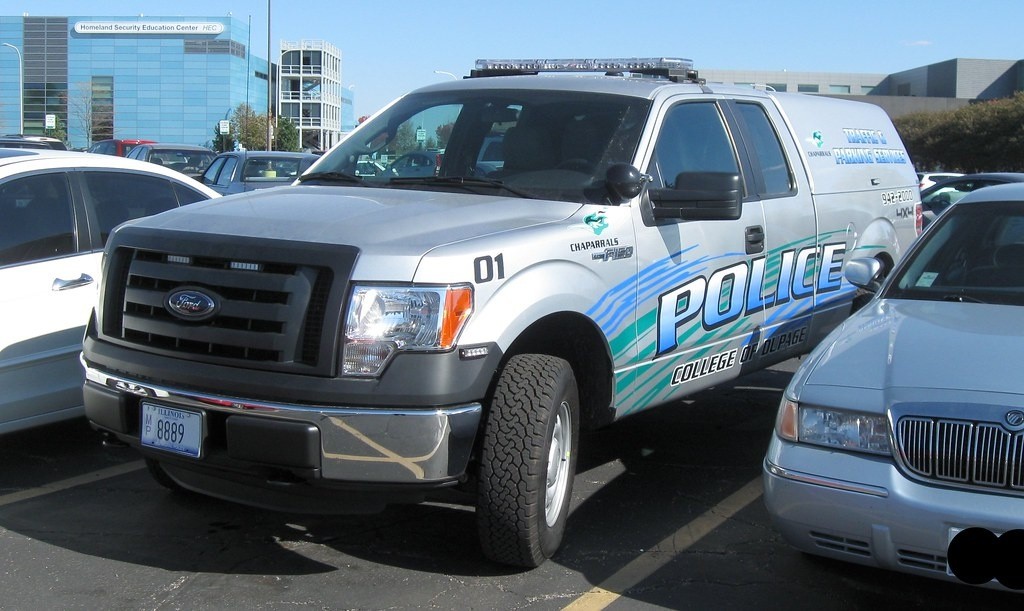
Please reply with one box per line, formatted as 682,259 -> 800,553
78,57 -> 924,574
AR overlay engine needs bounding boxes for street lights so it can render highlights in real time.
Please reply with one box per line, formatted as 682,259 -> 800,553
0,41 -> 24,133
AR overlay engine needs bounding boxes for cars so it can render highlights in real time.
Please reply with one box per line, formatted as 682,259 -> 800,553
473,132 -> 510,175
916,171 -> 978,205
0,134 -> 67,151
0,148 -> 241,436
761,182 -> 1024,595
124,142 -> 219,186
355,161 -> 401,176
377,150 -> 446,179
198,148 -> 325,197
920,173 -> 1023,232
83,139 -> 159,158
